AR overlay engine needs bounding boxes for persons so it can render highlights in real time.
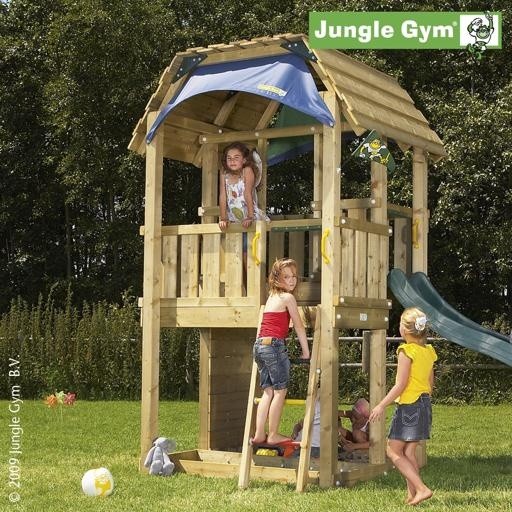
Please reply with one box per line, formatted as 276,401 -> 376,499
219,142 -> 271,282
291,388 -> 369,458
368,308 -> 437,505
251,258 -> 310,447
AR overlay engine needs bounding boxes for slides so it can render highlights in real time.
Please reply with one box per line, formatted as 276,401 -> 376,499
388,269 -> 512,369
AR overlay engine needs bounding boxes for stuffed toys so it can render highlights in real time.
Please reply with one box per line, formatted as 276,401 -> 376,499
144,437 -> 175,475
